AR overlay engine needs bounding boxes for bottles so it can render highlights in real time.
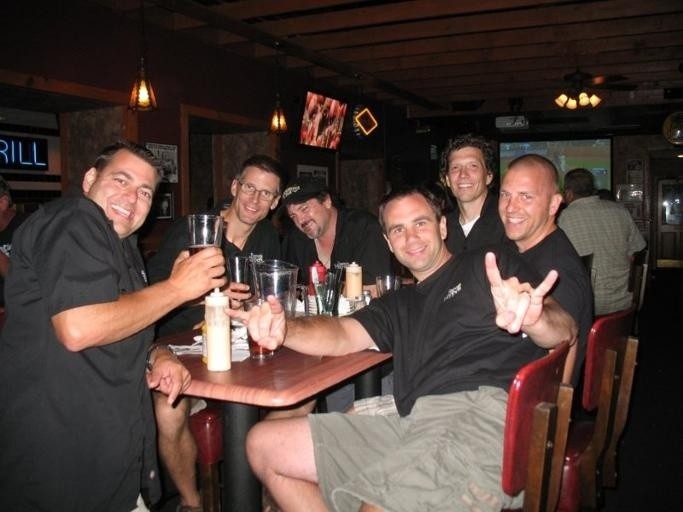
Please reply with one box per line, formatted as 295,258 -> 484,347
353,290 -> 370,310
203,287 -> 232,374
344,260 -> 363,298
306,260 -> 326,315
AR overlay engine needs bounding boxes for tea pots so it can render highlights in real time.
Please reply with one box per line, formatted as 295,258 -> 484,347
253,258 -> 297,315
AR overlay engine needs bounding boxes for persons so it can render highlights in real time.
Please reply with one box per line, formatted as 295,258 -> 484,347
0,176 -> 30,280
442,129 -> 509,256
283,171 -> 394,292
1,137 -> 229,512
556,164 -> 649,315
244,182 -> 581,512
146,151 -> 285,512
496,154 -> 593,387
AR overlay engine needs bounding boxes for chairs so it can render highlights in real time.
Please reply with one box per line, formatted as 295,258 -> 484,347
556,305 -> 635,510
355,341 -> 572,512
189,406 -> 225,512
629,250 -> 650,335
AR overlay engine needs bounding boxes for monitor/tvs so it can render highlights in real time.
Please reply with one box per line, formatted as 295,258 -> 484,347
296,88 -> 350,154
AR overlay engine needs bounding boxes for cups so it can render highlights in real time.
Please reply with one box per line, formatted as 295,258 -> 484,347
228,254 -> 249,299
243,301 -> 276,356
375,275 -> 402,297
312,281 -> 343,317
186,213 -> 223,259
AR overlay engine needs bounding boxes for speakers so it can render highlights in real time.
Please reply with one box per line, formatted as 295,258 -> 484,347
658,180 -> 683,232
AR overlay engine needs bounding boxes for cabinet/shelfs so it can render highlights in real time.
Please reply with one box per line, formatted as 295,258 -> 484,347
647,149 -> 683,298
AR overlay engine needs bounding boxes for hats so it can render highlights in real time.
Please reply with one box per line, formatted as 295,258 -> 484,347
276,178 -> 328,218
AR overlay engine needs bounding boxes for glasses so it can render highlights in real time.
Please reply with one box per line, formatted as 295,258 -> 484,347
235,178 -> 277,201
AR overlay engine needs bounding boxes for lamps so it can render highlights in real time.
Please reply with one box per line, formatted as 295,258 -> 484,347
554,71 -> 600,109
127,19 -> 159,112
264,43 -> 289,133
